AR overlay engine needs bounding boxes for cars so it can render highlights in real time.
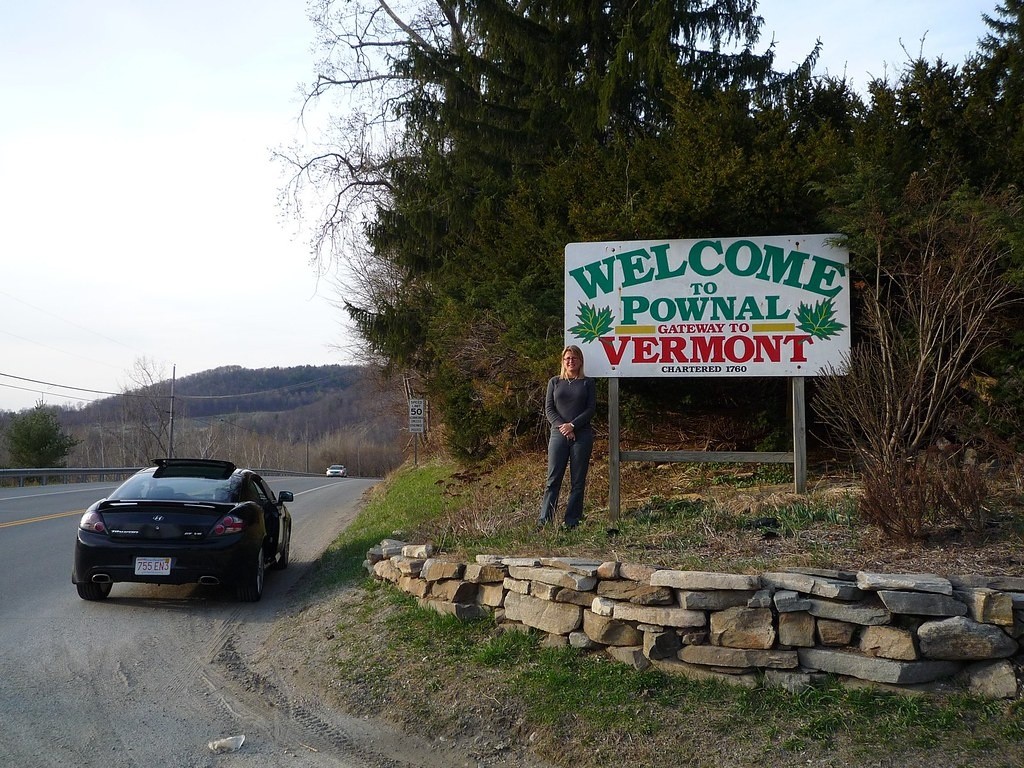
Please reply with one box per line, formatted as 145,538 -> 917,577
325,464 -> 348,478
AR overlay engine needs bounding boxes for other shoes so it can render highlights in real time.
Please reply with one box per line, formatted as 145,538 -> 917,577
531,525 -> 542,534
565,523 -> 575,533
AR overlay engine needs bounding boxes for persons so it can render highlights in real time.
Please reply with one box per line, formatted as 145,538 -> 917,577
536,345 -> 597,532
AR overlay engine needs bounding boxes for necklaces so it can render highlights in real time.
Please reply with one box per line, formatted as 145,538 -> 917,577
566,376 -> 578,386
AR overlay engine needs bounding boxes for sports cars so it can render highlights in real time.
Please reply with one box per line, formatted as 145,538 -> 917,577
70,454 -> 295,600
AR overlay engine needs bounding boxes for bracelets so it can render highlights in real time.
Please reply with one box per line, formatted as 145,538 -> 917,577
570,423 -> 575,428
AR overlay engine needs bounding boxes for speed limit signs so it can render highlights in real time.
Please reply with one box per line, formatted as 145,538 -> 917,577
409,399 -> 424,418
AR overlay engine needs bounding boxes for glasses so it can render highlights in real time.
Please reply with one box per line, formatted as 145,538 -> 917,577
563,357 -> 580,362
